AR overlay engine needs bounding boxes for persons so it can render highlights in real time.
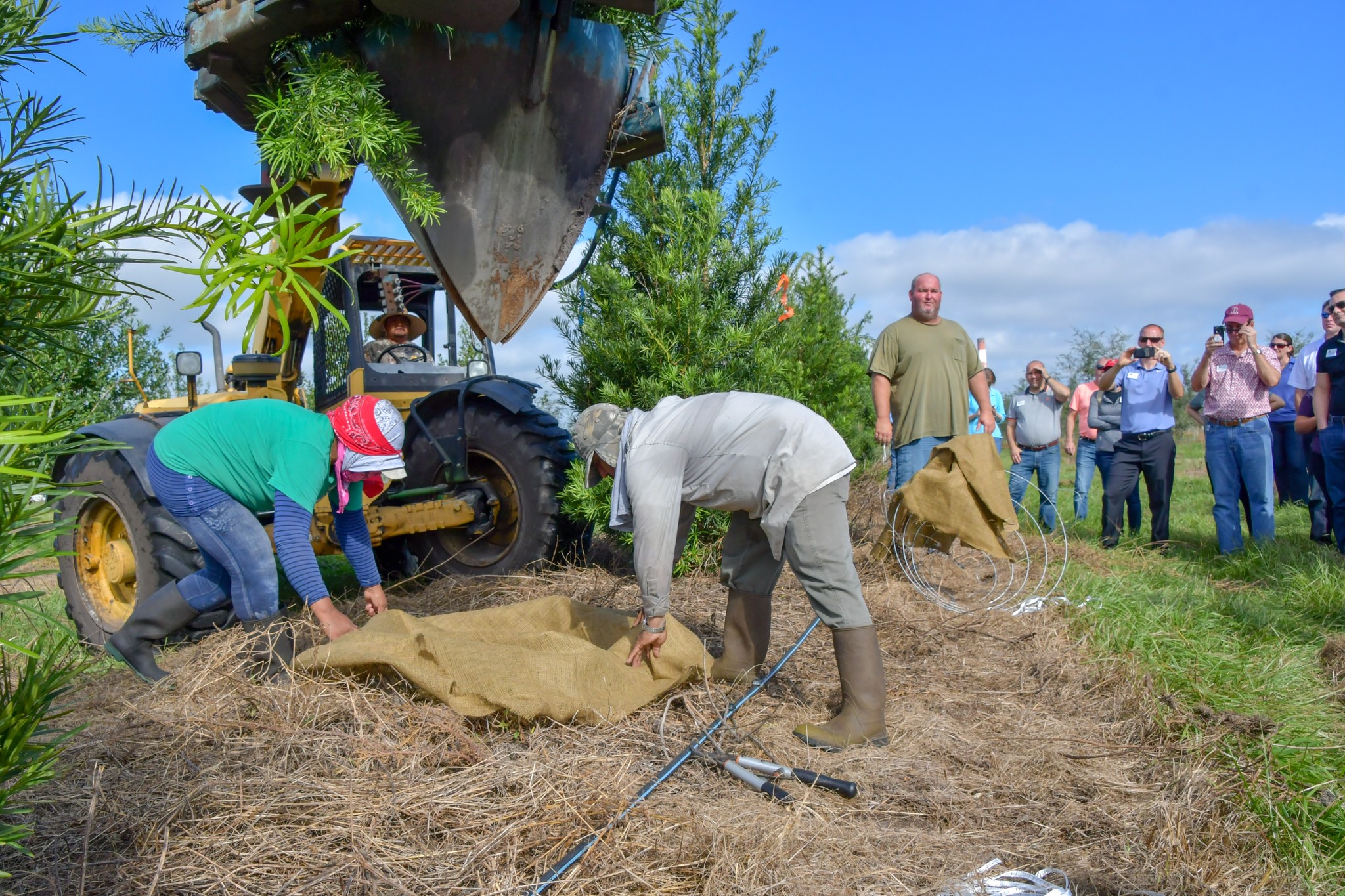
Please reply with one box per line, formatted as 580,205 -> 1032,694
1268,332 -> 1308,507
1190,304 -> 1283,555
1006,361 -> 1071,532
1064,358 -> 1111,522
865,272 -> 996,515
363,313 -> 435,364
103,392 -> 407,683
1097,324 -> 1184,550
571,390 -> 887,752
1293,385 -> 1333,544
968,368 -> 1006,454
1186,387 -> 1252,535
1088,358 -> 1141,536
1286,300 -> 1340,412
1310,284 -> 1345,555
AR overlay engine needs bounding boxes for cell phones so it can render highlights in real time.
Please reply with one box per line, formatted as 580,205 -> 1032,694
1133,346 -> 1154,358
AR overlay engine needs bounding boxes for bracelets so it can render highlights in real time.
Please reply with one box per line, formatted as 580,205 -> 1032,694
1045,376 -> 1052,383
1167,365 -> 1176,373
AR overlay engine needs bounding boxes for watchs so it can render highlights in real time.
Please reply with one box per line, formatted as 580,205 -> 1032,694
1253,348 -> 1263,355
641,618 -> 667,633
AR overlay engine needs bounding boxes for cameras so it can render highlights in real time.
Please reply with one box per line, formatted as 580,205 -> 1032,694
1214,325 -> 1224,347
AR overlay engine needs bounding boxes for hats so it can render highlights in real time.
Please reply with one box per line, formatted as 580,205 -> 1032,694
374,399 -> 408,480
571,403 -> 632,489
1221,303 -> 1254,324
1102,358 -> 1118,370
368,307 -> 427,341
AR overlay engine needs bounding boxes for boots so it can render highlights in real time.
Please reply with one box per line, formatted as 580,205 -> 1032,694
103,579 -> 202,691
792,622 -> 891,752
236,603 -> 301,685
686,588 -> 772,687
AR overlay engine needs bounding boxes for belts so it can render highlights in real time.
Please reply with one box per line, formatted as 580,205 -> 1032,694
1079,435 -> 1096,444
1206,414 -> 1266,426
1121,428 -> 1171,441
1017,440 -> 1059,451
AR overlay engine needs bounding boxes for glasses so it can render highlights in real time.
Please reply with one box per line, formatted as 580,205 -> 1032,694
1223,323 -> 1250,334
1096,365 -> 1105,370
1321,312 -> 1331,318
1269,343 -> 1288,348
1327,300 -> 1345,313
1139,336 -> 1163,343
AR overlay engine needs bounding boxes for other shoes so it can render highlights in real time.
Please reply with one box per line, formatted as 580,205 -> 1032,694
1310,533 -> 1335,548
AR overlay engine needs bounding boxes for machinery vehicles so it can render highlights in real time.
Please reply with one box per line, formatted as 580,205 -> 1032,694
55,0 -> 665,653
